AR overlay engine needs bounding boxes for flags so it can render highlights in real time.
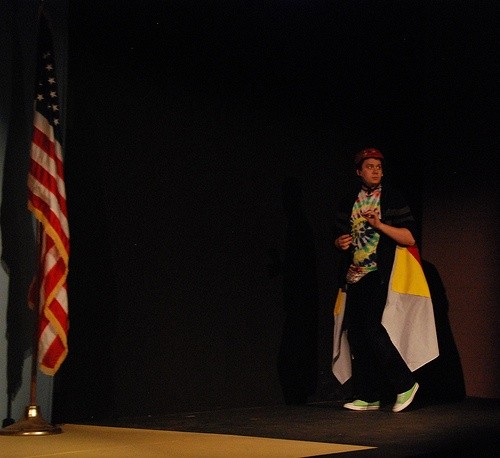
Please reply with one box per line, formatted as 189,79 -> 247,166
28,8 -> 71,379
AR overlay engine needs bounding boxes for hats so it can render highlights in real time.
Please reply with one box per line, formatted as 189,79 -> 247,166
353,149 -> 384,165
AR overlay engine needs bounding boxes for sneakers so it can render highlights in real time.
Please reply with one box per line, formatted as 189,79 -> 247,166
394,382 -> 419,411
343,399 -> 380,410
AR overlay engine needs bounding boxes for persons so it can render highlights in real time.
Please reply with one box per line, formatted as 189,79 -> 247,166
331,149 -> 440,412
